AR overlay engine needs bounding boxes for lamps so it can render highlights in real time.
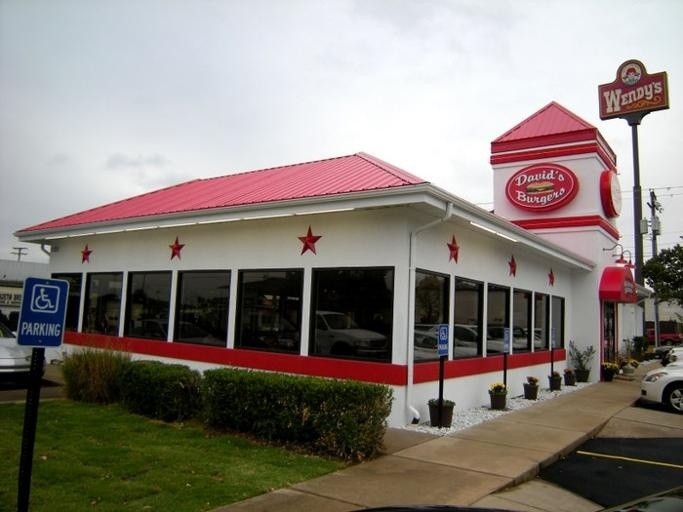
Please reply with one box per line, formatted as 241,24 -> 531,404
612,249 -> 637,273
603,240 -> 628,268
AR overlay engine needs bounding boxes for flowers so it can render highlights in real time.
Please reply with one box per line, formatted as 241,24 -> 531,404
600,360 -> 620,369
488,382 -> 509,393
524,374 -> 538,385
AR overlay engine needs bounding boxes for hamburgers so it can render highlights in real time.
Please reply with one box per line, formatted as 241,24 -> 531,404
527,182 -> 554,195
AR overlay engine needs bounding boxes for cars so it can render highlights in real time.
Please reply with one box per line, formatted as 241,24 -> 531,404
640,336 -> 683,417
131,318 -> 225,347
0,317 -> 46,384
413,317 -> 554,362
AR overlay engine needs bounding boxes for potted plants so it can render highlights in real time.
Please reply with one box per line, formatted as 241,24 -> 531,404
426,396 -> 455,428
565,368 -> 576,388
567,337 -> 595,386
548,371 -> 563,392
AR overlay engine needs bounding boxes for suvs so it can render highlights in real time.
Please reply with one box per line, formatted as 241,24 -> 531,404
300,308 -> 389,360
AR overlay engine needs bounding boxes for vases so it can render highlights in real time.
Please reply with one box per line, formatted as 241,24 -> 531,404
490,393 -> 508,410
602,369 -> 616,382
522,384 -> 540,399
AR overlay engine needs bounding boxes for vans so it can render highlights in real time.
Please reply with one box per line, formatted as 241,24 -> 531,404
241,310 -> 300,351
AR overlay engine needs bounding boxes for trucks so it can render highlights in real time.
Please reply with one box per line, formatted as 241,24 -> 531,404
645,328 -> 683,346
